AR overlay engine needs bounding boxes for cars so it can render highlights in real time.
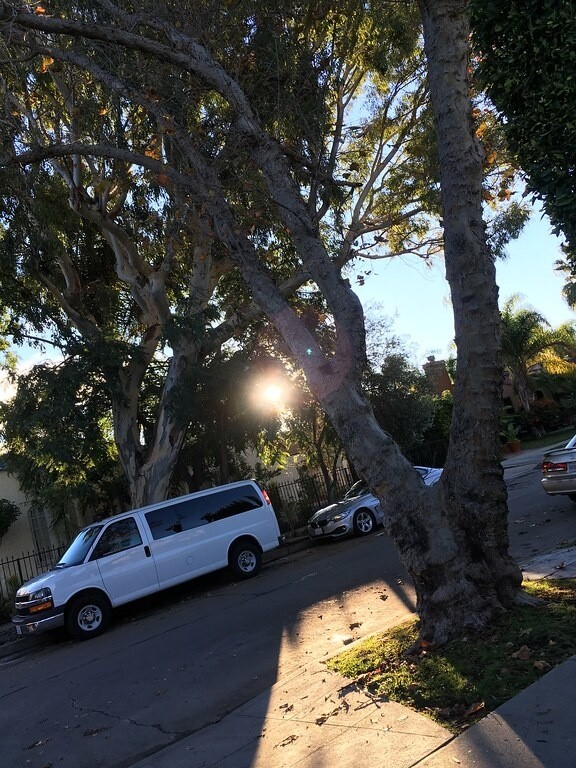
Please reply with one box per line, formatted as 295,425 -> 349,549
309,466 -> 445,540
541,432 -> 575,502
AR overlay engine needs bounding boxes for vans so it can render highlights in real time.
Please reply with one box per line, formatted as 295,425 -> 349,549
13,478 -> 286,639
